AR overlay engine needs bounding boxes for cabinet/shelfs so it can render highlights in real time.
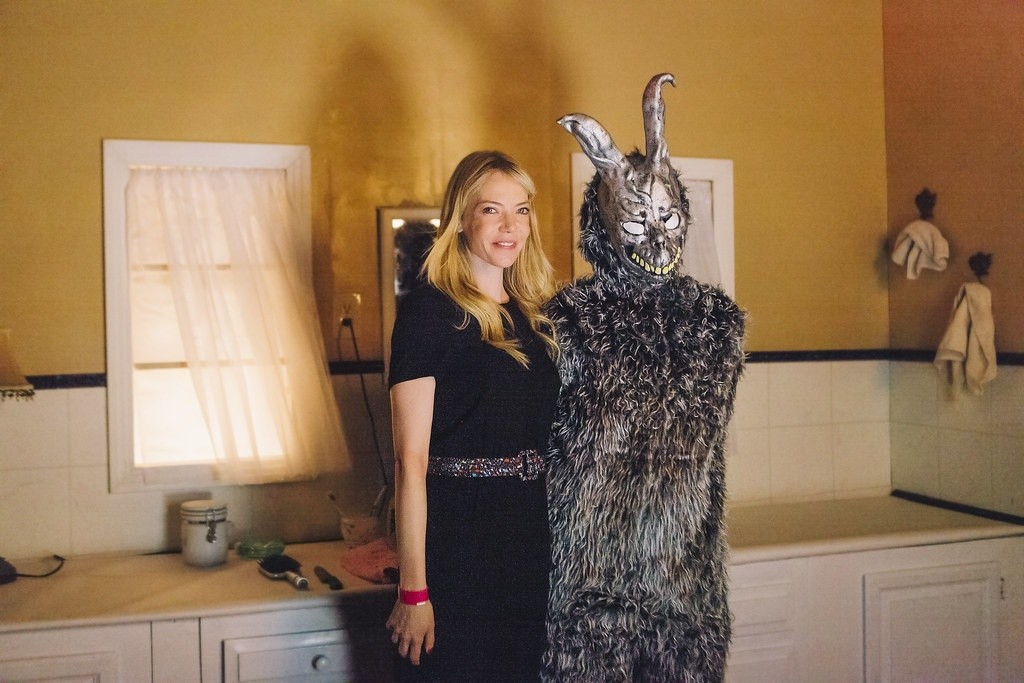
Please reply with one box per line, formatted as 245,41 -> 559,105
0,534 -> 1024,683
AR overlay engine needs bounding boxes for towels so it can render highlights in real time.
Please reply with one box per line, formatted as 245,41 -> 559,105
933,282 -> 997,397
891,220 -> 949,280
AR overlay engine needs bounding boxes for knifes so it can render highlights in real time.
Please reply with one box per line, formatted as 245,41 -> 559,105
315,566 -> 343,590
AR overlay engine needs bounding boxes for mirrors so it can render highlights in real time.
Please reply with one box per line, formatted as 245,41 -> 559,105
376,205 -> 442,385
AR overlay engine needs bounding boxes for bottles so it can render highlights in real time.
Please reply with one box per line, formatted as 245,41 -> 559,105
180,499 -> 229,566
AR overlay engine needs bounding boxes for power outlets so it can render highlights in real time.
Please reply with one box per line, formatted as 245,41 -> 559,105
333,293 -> 363,337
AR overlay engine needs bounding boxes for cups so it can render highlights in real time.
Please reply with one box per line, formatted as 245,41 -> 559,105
342,512 -> 377,549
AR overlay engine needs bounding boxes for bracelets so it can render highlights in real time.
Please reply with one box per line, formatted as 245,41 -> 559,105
398,585 -> 428,605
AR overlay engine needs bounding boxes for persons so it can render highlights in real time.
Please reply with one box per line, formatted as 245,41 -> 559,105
387,150 -> 561,683
